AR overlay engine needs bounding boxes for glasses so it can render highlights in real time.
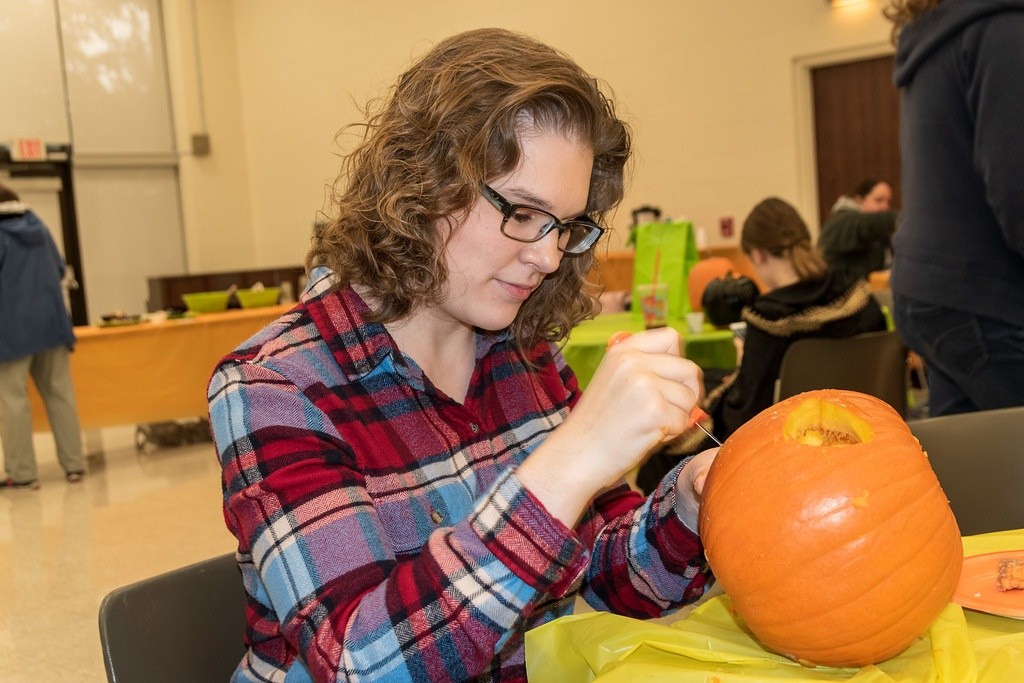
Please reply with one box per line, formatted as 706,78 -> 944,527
477,179 -> 604,257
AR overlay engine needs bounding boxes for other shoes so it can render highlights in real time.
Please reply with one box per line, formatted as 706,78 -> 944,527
63,470 -> 85,481
0,478 -> 41,489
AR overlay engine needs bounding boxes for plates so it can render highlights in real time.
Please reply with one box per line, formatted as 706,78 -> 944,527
950,550 -> 1024,620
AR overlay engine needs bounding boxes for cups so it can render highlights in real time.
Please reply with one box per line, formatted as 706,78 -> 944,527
638,282 -> 670,330
685,311 -> 704,333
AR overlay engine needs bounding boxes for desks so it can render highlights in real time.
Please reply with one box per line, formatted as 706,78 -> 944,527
25,301 -> 298,433
520,527 -> 1024,683
535,310 -> 747,399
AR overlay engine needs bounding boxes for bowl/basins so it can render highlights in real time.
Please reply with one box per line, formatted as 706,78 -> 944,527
181,289 -> 235,314
234,287 -> 285,307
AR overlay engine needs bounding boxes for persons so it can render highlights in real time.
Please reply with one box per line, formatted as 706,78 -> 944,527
884,0 -> 1024,419
203,28 -> 721,682
634,200 -> 889,500
1,184 -> 86,489
815,177 -> 899,280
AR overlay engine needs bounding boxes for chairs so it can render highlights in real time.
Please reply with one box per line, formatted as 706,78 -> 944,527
97,550 -> 251,682
762,332 -> 1023,540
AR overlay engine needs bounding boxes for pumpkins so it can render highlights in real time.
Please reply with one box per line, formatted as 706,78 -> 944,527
688,257 -> 740,313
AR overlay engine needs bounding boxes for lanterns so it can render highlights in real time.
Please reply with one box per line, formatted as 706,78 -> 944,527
698,389 -> 963,667
